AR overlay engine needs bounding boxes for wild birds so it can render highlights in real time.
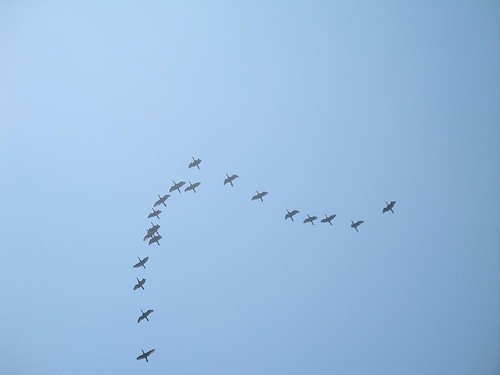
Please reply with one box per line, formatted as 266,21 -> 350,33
382,201 -> 397,214
143,222 -> 163,246
351,220 -> 364,232
285,209 -> 300,221
133,256 -> 149,270
147,193 -> 171,219
137,309 -> 154,324
133,277 -> 146,291
321,214 -> 336,226
188,157 -> 202,169
251,190 -> 269,202
169,180 -> 201,194
224,173 -> 239,187
303,214 -> 318,226
137,348 -> 157,363
